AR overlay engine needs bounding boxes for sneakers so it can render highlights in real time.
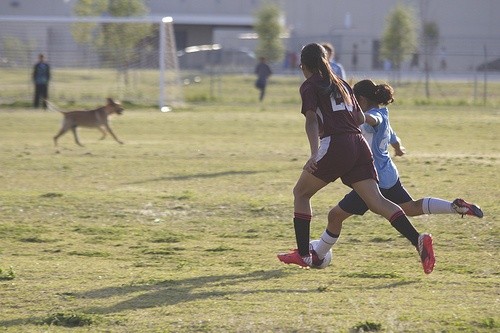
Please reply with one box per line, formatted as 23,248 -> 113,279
418,233 -> 436,274
277,249 -> 312,270
311,250 -> 325,266
451,198 -> 483,218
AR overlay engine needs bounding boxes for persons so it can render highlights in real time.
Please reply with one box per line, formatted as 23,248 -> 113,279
277,43 -> 484,273
34,53 -> 51,111
254,57 -> 272,102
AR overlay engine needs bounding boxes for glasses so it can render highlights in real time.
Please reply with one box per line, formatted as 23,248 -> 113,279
299,63 -> 308,68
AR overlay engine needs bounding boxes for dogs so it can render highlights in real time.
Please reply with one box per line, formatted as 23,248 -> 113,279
41,93 -> 126,150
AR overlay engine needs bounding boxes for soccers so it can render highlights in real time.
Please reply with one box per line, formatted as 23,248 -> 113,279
309,241 -> 330,270
